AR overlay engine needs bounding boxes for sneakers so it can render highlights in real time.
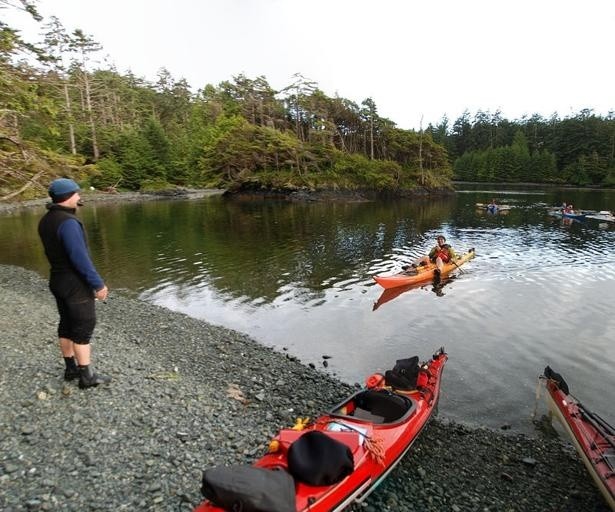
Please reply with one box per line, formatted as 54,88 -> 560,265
402,265 -> 410,270
65,364 -> 81,380
434,269 -> 441,275
80,374 -> 112,389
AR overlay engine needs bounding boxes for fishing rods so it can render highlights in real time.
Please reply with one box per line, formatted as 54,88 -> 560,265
552,381 -> 615,476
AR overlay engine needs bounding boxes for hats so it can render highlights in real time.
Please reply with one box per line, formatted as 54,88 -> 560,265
49,179 -> 81,203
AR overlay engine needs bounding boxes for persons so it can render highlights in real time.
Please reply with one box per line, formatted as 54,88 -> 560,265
561,203 -> 574,214
488,198 -> 498,213
401,235 -> 457,276
37,177 -> 113,390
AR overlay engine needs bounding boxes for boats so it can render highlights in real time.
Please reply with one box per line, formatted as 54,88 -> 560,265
196,347 -> 447,512
488,204 -> 497,214
536,364 -> 615,508
372,247 -> 474,290
374,276 -> 450,312
561,212 -> 585,220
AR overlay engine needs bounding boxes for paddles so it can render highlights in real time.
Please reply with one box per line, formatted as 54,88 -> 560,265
436,246 -> 468,275
